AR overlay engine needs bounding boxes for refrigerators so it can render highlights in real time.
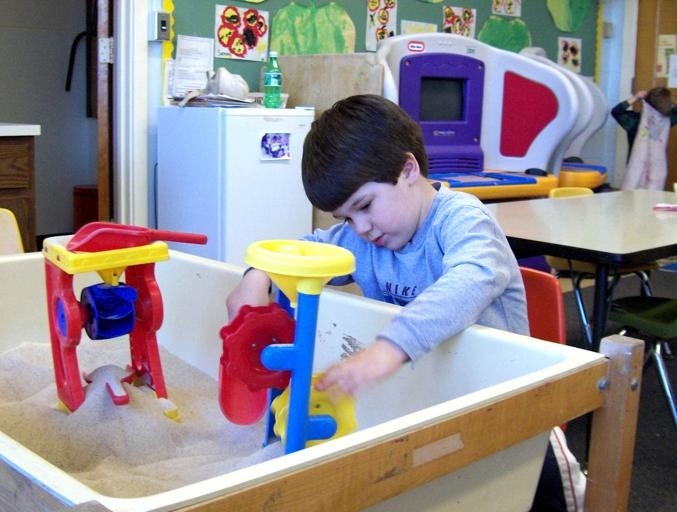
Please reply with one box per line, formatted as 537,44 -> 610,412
155,104 -> 312,266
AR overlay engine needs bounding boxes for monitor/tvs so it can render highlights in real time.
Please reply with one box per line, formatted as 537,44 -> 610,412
420,76 -> 463,121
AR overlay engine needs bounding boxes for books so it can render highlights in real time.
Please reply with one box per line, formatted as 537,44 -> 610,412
173,92 -> 258,109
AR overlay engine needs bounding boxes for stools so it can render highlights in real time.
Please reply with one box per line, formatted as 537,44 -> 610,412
602,291 -> 676,436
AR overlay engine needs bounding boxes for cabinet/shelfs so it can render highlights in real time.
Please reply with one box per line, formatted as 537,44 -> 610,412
0,123 -> 44,257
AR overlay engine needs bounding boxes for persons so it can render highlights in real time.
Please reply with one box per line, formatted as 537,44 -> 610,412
270,136 -> 285,158
227,93 -> 589,511
608,83 -> 676,190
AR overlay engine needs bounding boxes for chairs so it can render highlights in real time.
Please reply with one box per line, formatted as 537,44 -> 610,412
0,208 -> 24,259
516,263 -> 571,436
541,182 -> 669,355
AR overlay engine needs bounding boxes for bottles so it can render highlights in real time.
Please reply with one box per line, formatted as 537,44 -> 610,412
262,52 -> 283,109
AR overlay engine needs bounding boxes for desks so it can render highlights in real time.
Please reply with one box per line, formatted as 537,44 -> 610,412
476,189 -> 676,350
